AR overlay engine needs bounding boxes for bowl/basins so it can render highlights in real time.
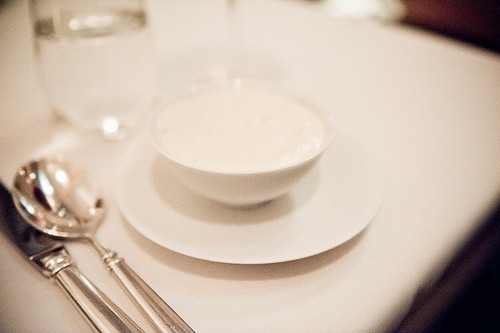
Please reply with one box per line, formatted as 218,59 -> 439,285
147,80 -> 336,208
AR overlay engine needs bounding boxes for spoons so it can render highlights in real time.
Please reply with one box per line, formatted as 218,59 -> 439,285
12,159 -> 197,333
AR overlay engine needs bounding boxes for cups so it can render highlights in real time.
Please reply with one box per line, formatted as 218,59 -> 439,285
28,0 -> 149,127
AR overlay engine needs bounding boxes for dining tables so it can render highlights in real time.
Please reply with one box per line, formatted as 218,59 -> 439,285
0,0 -> 499,333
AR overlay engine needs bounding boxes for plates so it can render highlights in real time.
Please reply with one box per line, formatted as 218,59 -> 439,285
114,138 -> 379,266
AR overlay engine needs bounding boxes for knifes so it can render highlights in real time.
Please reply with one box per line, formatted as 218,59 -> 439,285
0,182 -> 146,333
